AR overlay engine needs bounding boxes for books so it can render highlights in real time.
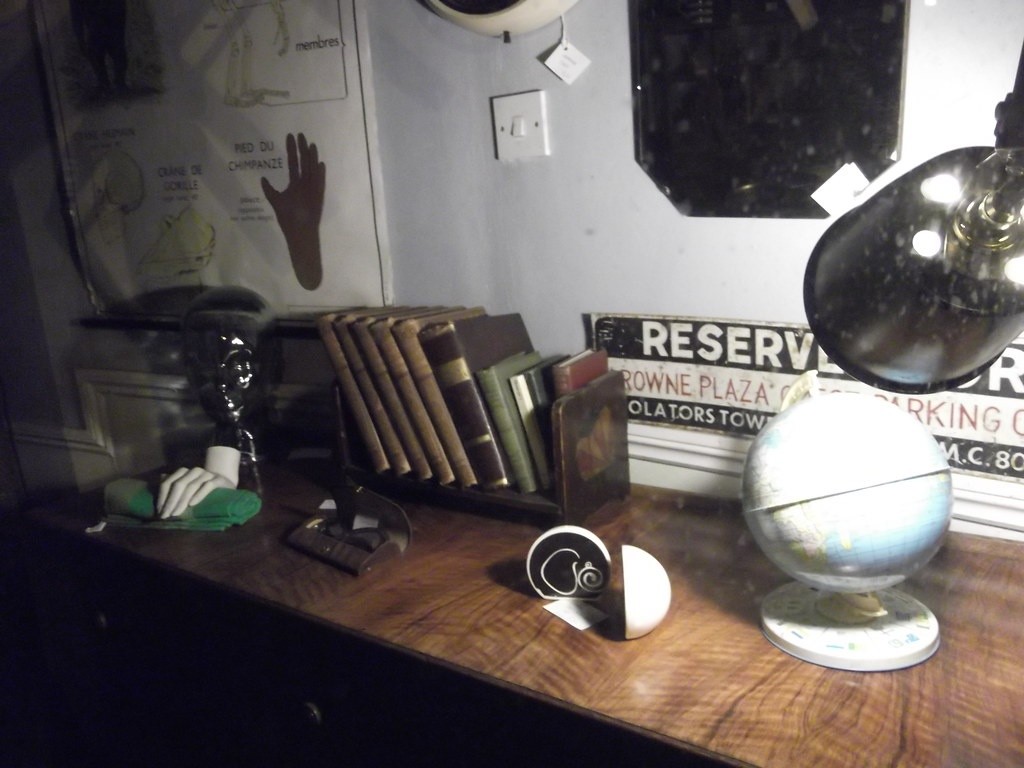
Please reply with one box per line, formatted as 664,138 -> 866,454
313,305 -> 608,495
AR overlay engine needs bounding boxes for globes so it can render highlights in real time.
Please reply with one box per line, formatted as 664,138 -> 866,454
740,369 -> 953,673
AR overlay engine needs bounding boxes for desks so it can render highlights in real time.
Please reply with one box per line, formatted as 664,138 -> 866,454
1,484 -> 1024,768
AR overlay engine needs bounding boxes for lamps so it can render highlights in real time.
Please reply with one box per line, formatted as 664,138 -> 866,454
804,40 -> 1024,396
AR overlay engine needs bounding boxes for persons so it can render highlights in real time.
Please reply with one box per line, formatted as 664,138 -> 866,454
157,446 -> 242,519
180,286 -> 286,492
